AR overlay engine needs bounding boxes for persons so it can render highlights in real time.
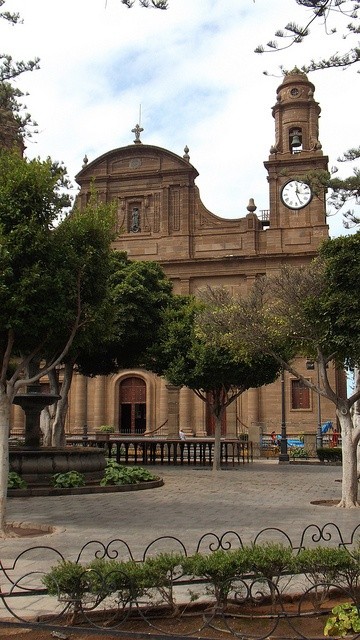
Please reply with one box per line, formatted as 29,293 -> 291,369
271,431 -> 278,444
179,429 -> 185,440
